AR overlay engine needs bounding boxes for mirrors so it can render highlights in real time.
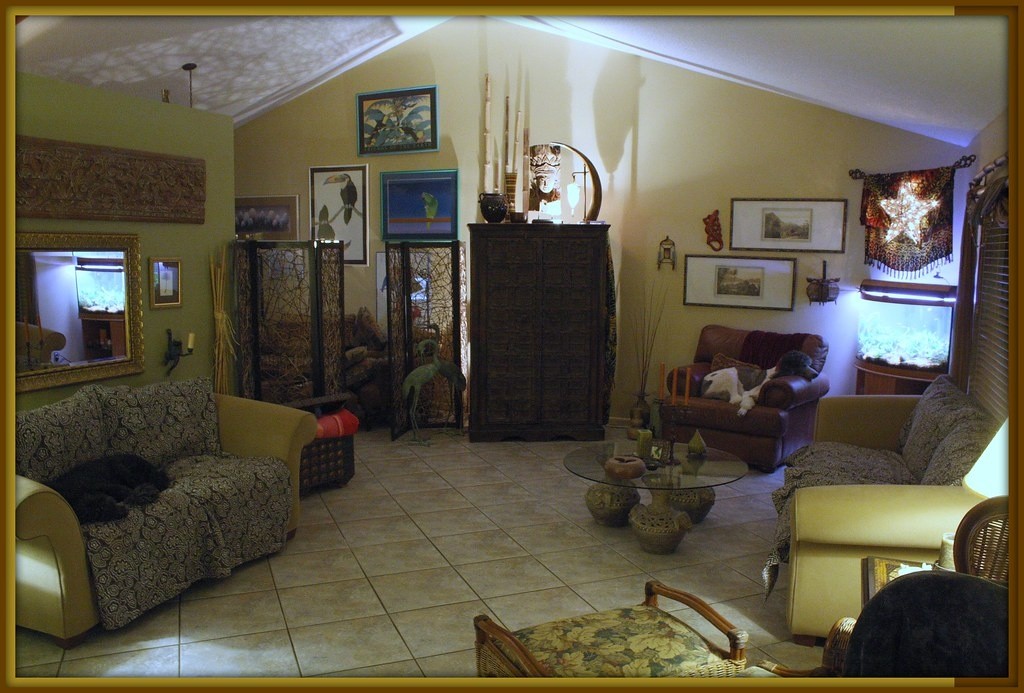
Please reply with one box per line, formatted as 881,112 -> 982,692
15,231 -> 145,392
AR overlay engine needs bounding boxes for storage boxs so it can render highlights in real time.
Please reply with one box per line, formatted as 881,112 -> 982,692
74,266 -> 127,319
852,277 -> 957,381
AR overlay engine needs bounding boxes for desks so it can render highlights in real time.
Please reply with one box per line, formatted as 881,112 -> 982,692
562,440 -> 749,556
861,555 -> 938,611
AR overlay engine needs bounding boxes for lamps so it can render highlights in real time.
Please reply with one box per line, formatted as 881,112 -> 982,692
656,236 -> 678,270
962,416 -> 1010,498
550,141 -> 604,225
806,260 -> 841,307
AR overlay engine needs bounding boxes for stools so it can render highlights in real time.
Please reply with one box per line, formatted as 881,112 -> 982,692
473,579 -> 749,679
295,407 -> 359,500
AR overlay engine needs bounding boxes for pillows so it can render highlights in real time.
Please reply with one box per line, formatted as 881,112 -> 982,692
16,375 -> 223,484
900,375 -> 1002,487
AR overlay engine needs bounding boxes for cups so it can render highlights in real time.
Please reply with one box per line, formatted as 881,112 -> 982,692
938,531 -> 955,571
511,211 -> 527,223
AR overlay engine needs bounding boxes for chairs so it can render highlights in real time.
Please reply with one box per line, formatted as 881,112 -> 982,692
953,496 -> 1009,584
732,579 -> 858,679
843,571 -> 1010,679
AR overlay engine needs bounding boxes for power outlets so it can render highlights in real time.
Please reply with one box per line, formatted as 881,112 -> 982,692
53,351 -> 60,362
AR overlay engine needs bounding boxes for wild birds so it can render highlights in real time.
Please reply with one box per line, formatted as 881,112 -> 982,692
401,322 -> 466,448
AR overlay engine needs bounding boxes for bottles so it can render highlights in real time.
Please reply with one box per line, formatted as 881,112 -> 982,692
477,192 -> 508,223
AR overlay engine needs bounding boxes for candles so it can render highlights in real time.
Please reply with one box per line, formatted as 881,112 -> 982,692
657,361 -> 692,409
187,332 -> 195,349
939,532 -> 957,572
23,313 -> 44,343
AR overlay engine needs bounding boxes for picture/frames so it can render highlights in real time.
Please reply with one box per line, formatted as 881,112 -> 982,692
149,256 -> 185,310
355,84 -> 440,157
729,197 -> 847,254
308,163 -> 370,267
375,249 -> 432,334
682,254 -> 797,312
380,168 -> 460,243
234,194 -> 301,243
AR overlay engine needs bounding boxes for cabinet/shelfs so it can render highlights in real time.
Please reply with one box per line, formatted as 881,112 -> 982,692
78,317 -> 127,361
853,364 -> 934,396
467,222 -> 612,443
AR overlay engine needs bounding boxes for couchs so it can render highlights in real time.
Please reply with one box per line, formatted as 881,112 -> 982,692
660,324 -> 832,474
762,373 -> 1003,649
15,321 -> 66,374
239,311 -> 446,432
15,374 -> 317,650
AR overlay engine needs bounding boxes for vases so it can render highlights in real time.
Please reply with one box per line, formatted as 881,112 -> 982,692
630,392 -> 651,428
505,171 -> 518,223
478,192 -> 511,223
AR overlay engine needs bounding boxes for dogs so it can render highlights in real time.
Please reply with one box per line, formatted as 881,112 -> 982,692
699,350 -> 819,418
43,456 -> 171,522
